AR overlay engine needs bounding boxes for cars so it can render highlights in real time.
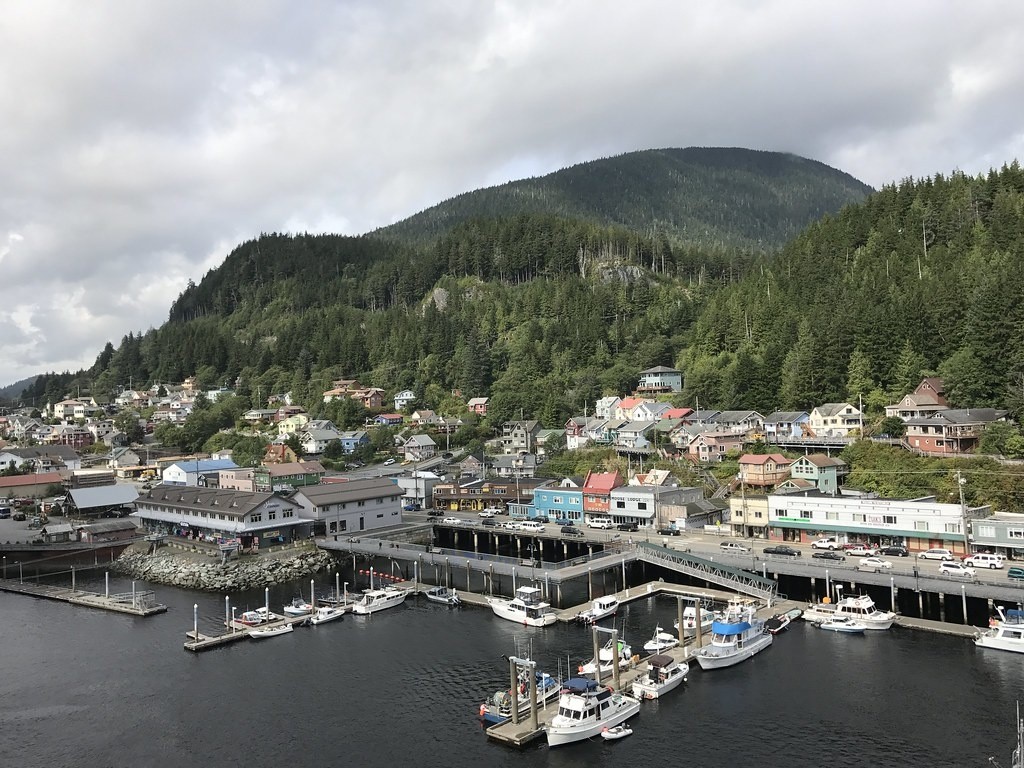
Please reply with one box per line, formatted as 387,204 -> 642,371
404,504 -> 638,536
718,538 -> 1024,580
657,527 -> 680,536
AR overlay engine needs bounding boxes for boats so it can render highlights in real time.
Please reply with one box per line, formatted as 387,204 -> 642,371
574,595 -> 619,624
318,588 -> 348,607
248,623 -> 294,638
283,597 -> 312,615
485,585 -> 557,626
479,635 -> 564,723
234,607 -> 276,626
311,606 -> 346,624
801,588 -> 898,632
546,678 -> 640,746
353,587 -> 408,614
425,586 -> 461,605
578,617 -> 633,679
972,602 -> 1024,652
631,600 -> 775,699
